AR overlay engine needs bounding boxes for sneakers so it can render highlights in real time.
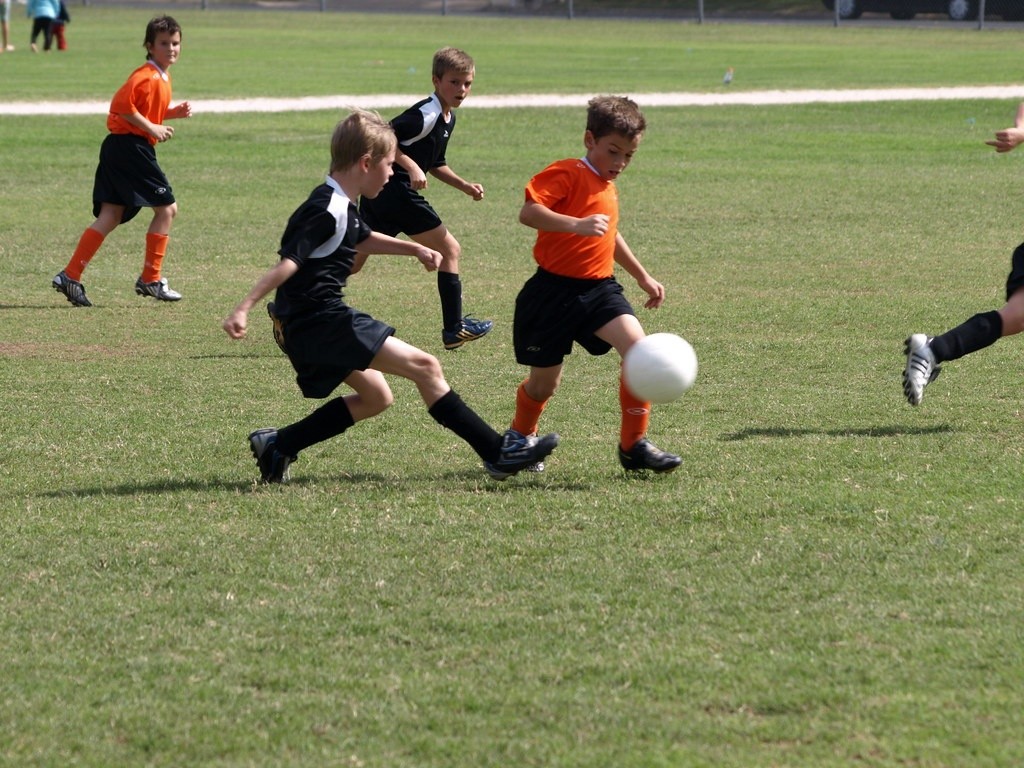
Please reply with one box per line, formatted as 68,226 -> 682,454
267,302 -> 287,354
902,333 -> 942,405
135,276 -> 182,302
442,314 -> 493,350
52,270 -> 93,307
510,426 -> 545,472
619,437 -> 683,471
483,429 -> 561,481
248,428 -> 291,484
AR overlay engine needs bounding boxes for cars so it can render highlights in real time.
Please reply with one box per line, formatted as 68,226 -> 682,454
822,0 -> 1024,22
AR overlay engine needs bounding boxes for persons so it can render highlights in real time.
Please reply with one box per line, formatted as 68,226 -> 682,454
25,0 -> 61,52
0,0 -> 15,52
222,108 -> 559,483
52,0 -> 70,50
508,95 -> 681,474
349,48 -> 495,349
52,15 -> 193,308
901,98 -> 1024,406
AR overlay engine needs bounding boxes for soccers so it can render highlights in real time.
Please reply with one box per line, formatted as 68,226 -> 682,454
626,332 -> 699,404
